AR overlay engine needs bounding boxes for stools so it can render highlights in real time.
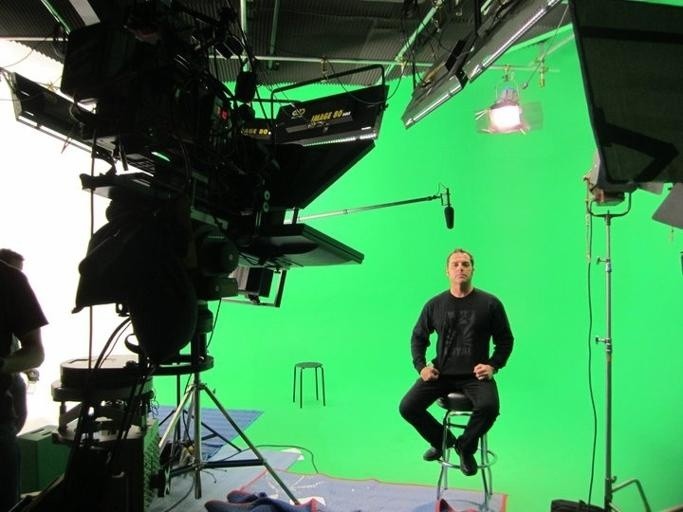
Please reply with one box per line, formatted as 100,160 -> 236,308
293,362 -> 325,408
437,391 -> 496,510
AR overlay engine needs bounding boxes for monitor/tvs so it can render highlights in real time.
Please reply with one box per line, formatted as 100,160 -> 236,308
274,83 -> 390,144
401,0 -> 562,129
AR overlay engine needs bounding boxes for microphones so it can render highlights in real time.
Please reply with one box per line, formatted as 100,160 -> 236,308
443,188 -> 456,229
157,375 -> 300,507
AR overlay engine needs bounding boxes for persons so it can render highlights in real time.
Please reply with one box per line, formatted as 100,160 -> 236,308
0,260 -> 46,512
398,248 -> 515,476
0,247 -> 40,433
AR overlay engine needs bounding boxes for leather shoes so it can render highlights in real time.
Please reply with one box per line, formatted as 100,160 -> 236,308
424,432 -> 456,461
454,439 -> 477,476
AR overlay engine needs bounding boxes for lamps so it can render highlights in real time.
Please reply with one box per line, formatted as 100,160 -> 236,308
475,65 -> 542,134
